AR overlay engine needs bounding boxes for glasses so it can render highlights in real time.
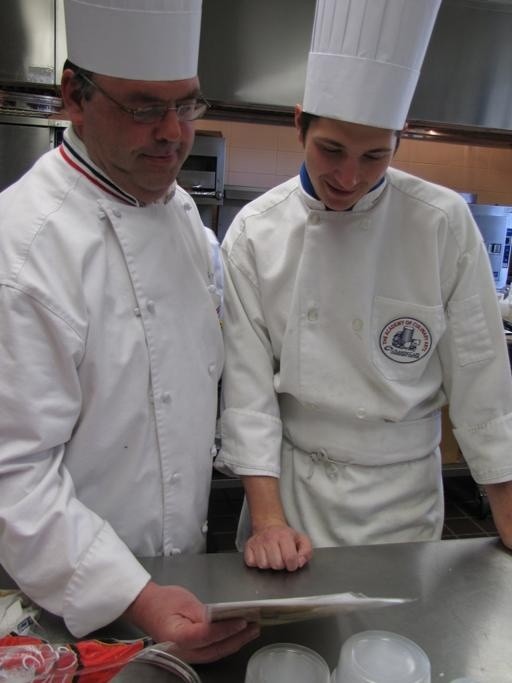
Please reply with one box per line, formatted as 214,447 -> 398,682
69,67 -> 211,125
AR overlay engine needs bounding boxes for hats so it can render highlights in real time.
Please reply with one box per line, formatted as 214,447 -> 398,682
302,0 -> 443,132
64,0 -> 203,83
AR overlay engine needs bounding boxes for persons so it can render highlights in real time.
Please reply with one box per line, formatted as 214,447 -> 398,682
0,60 -> 262,666
214,101 -> 512,573
